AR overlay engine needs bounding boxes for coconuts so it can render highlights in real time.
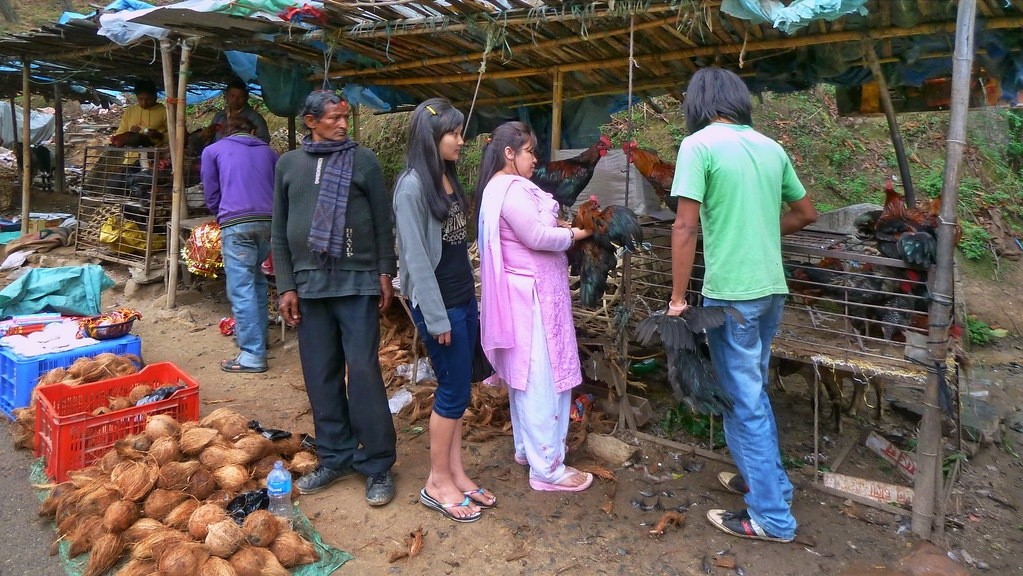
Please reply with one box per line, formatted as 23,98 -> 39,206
8,352 -> 322,575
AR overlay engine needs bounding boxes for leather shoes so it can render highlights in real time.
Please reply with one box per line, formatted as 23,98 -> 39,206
365,469 -> 395,505
293,466 -> 361,495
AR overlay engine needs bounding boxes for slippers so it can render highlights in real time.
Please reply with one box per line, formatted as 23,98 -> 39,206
463,488 -> 498,509
529,466 -> 594,492
514,445 -> 570,466
717,471 -> 793,508
420,486 -> 482,522
707,508 -> 796,543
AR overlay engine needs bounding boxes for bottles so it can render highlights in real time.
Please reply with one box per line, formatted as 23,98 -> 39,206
267,461 -> 294,534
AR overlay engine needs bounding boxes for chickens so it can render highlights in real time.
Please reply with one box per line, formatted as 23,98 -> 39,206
531,136 -> 611,223
107,124 -> 222,230
622,141 -> 700,224
563,195 -> 645,310
783,181 -> 963,352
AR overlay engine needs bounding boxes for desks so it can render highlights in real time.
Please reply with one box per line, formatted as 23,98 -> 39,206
165,215 -> 287,343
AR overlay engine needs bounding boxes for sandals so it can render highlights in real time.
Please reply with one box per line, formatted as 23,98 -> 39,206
219,359 -> 268,372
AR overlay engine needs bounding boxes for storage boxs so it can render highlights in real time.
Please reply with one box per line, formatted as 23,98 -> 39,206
0,332 -> 141,422
35,361 -> 200,484
28,219 -> 62,234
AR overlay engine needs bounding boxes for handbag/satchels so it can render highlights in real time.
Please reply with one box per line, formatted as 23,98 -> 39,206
468,320 -> 499,383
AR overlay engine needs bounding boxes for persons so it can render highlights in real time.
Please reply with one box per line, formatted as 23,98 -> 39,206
388,95 -> 499,522
199,113 -> 279,373
109,76 -> 169,163
474,120 -> 595,492
208,77 -> 271,143
667,65 -> 822,544
269,87 -> 401,508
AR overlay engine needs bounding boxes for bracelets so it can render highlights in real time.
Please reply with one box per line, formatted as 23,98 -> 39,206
668,299 -> 688,311
